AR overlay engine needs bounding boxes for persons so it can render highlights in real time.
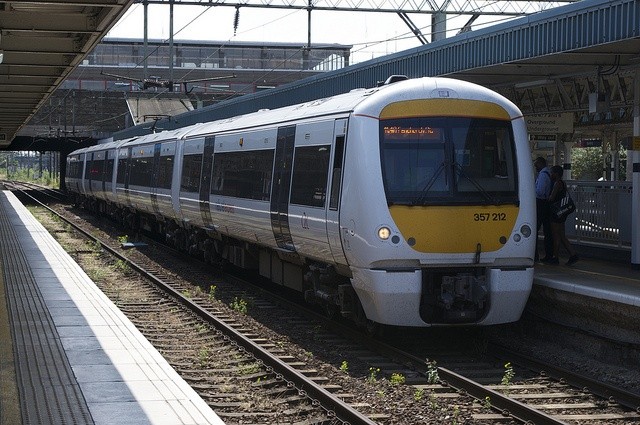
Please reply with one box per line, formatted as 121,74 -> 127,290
548,165 -> 579,265
534,157 -> 553,262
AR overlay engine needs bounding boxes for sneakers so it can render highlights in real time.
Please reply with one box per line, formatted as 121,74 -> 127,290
540,257 -> 553,263
548,256 -> 559,265
565,254 -> 581,266
535,254 -> 539,261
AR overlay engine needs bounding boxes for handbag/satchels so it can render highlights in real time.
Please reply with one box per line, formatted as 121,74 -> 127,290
548,196 -> 575,219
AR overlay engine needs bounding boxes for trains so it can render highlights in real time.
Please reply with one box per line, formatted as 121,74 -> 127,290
63,74 -> 541,328
